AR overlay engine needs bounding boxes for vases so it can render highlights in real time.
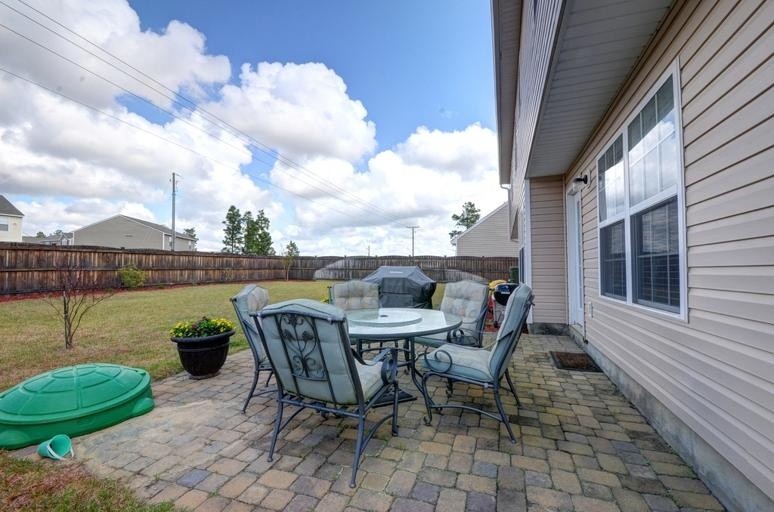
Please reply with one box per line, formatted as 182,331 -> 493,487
170,329 -> 235,379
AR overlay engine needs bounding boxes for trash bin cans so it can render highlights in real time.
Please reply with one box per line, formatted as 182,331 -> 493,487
491,282 -> 519,328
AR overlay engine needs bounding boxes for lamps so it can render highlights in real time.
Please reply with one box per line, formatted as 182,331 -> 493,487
571,170 -> 591,191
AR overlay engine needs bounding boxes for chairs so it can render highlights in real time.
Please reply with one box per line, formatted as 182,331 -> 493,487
249,298 -> 400,488
413,283 -> 535,443
229,283 -> 278,414
328,279 -> 400,373
404,279 -> 489,397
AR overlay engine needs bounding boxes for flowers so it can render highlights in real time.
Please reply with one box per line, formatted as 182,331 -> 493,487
167,315 -> 235,337
